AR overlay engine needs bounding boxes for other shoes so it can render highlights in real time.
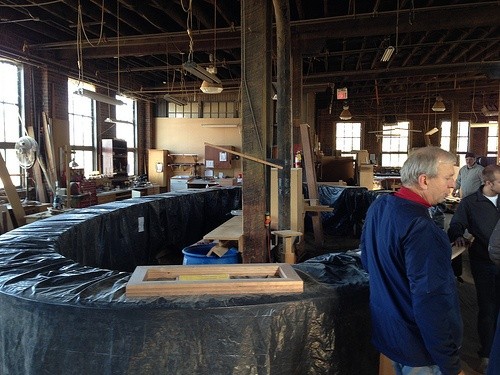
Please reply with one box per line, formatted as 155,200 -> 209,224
480,357 -> 489,371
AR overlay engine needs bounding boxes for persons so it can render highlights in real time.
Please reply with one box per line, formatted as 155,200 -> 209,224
452,151 -> 485,199
485,218 -> 500,375
448,164 -> 500,369
360,146 -> 465,375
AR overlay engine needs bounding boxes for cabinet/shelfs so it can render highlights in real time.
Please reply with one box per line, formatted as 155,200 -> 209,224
101,139 -> 127,175
204,145 -> 233,169
147,149 -> 168,188
168,151 -> 197,177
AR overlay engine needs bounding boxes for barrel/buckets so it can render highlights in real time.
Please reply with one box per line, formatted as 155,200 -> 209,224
182,246 -> 237,265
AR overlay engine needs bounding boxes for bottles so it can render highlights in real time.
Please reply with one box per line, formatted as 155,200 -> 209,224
294,154 -> 301,168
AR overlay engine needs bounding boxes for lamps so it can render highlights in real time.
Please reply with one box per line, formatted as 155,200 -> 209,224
73,0 -> 500,136
